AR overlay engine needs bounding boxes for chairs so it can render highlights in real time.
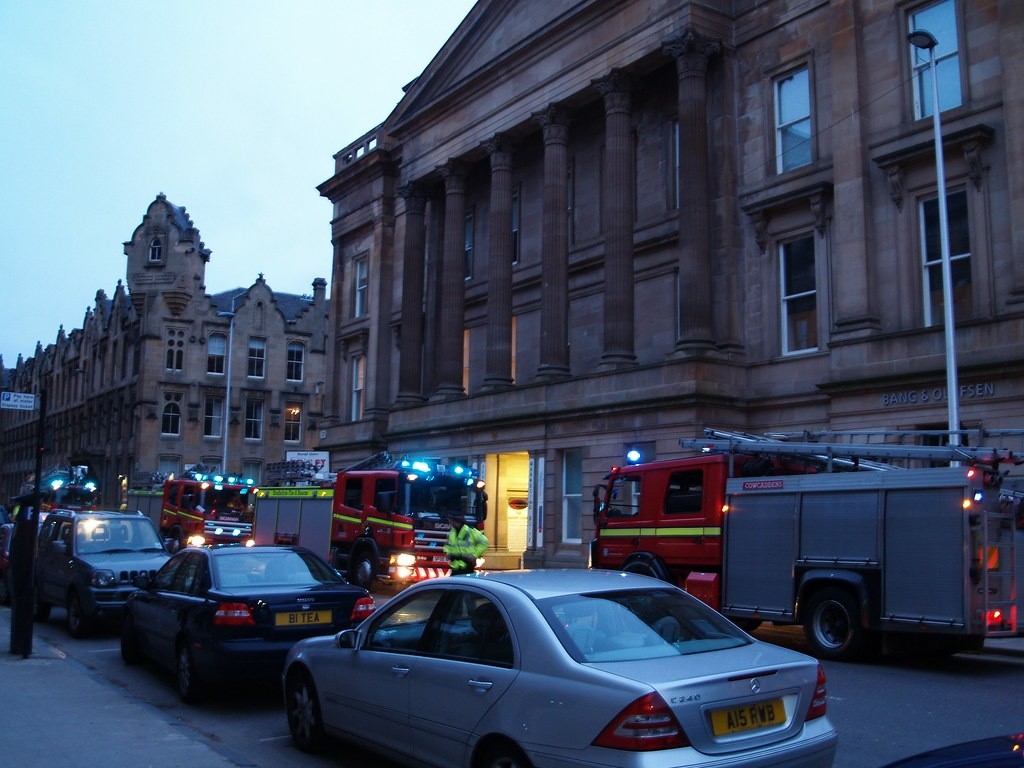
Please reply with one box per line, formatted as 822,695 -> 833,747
454,603 -> 682,662
62,524 -> 135,554
219,570 -> 316,586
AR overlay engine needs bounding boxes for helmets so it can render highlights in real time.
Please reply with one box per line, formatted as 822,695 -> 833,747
447,510 -> 465,522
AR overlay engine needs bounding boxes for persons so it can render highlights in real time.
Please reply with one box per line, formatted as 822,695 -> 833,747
445,511 -> 489,617
10,503 -> 20,523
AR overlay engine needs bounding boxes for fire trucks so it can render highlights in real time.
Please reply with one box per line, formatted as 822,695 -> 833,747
117,463 -> 258,553
10,465 -> 100,533
587,424 -> 1024,664
251,451 -> 489,593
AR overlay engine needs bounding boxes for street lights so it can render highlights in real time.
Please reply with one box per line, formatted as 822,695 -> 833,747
216,310 -> 236,475
905,29 -> 962,468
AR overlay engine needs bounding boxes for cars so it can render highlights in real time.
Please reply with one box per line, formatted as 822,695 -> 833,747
0,523 -> 43,603
281,568 -> 838,768
116,544 -> 376,709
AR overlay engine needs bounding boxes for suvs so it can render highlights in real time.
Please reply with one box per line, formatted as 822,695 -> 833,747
34,508 -> 194,640
